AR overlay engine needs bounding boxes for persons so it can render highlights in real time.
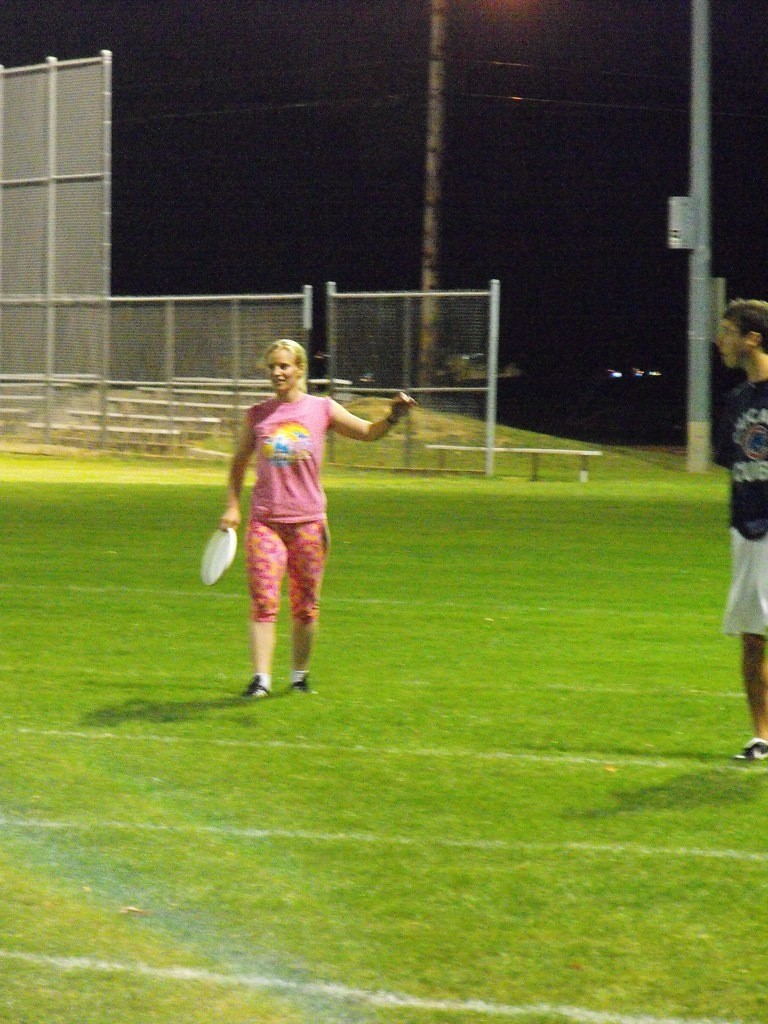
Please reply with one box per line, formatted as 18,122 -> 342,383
222,340 -> 417,698
711,299 -> 768,760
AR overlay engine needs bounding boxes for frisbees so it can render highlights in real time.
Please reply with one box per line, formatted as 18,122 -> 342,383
199,527 -> 238,586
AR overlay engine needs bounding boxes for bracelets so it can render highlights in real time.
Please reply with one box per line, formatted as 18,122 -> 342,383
388,416 -> 398,425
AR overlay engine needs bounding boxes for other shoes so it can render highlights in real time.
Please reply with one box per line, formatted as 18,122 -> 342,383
241,676 -> 270,698
732,737 -> 768,761
290,678 -> 318,696
